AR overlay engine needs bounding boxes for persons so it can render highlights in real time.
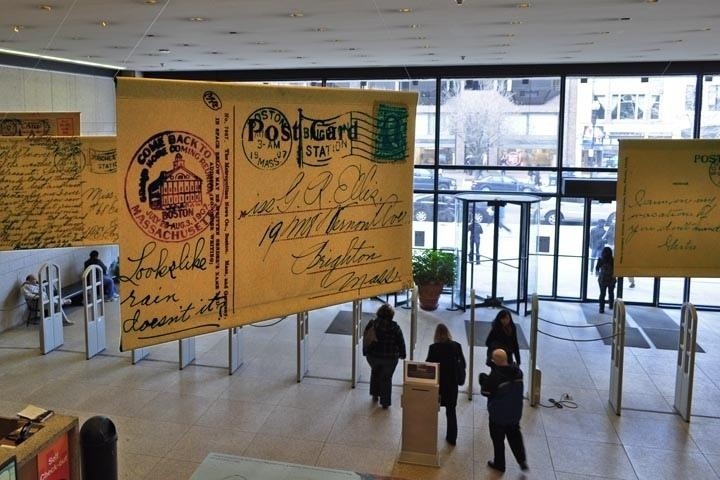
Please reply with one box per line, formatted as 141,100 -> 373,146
20,274 -> 75,326
526,163 -> 542,187
467,214 -> 483,265
499,157 -> 506,176
84,250 -> 119,302
363,304 -> 529,471
590,215 -> 634,314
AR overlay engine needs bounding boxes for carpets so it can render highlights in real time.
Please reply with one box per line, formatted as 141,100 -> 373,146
324,311 -> 373,336
464,319 -> 529,350
580,302 -> 705,353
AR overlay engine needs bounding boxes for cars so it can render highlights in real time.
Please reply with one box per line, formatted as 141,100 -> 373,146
413,169 -> 616,225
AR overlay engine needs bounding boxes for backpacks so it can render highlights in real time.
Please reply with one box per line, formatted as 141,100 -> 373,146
598,262 -> 615,286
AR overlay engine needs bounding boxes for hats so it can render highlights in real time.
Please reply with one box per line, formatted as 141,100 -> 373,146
490,348 -> 509,366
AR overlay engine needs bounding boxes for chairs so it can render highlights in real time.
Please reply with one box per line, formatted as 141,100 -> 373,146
25,297 -> 40,328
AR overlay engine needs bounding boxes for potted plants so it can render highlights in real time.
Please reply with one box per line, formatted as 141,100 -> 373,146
412,249 -> 458,311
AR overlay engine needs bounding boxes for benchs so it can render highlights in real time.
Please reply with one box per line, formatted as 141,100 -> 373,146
61,274 -> 117,299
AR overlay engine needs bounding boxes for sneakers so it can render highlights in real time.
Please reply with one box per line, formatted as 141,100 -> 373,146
63,292 -> 118,325
488,459 -> 506,472
373,396 -> 389,409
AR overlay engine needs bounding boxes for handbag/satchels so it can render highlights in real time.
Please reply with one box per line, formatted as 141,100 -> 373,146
363,322 -> 378,355
453,345 -> 467,386
